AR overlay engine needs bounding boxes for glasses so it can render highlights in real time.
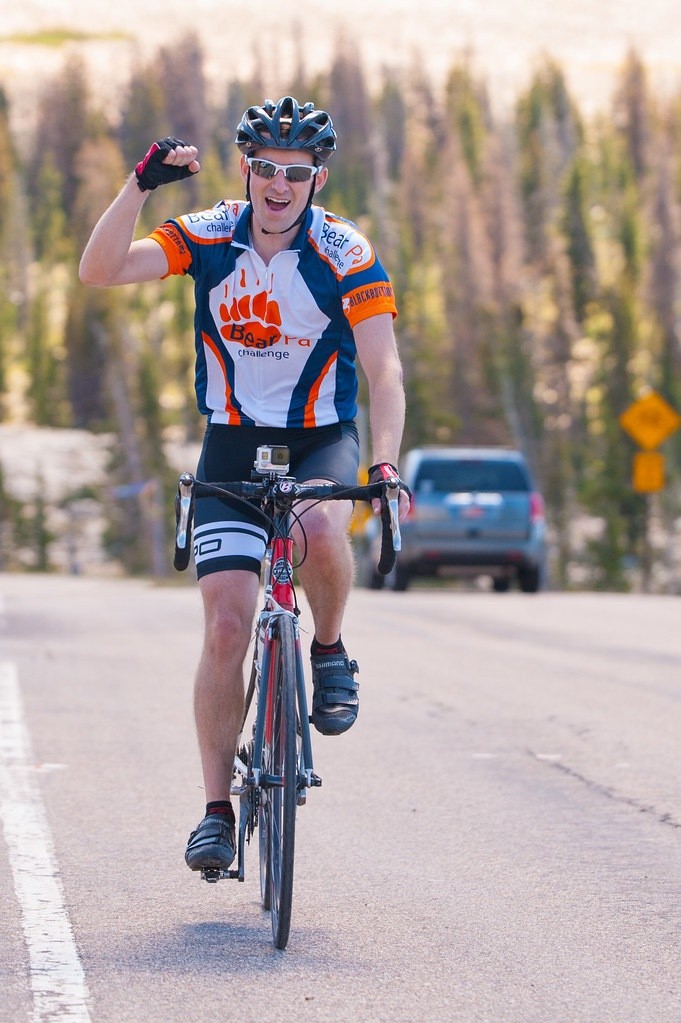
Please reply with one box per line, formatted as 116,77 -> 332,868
245,156 -> 322,182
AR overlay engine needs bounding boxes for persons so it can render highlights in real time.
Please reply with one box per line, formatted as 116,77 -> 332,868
76,92 -> 412,874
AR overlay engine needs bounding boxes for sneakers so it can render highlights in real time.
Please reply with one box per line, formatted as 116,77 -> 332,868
310,653 -> 359,735
185,814 -> 236,869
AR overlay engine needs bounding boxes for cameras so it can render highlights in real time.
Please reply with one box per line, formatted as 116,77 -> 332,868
254,444 -> 290,476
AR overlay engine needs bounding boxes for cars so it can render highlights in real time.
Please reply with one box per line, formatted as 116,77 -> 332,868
379,445 -> 548,596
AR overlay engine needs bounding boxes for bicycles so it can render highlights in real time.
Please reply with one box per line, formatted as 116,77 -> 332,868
170,445 -> 407,955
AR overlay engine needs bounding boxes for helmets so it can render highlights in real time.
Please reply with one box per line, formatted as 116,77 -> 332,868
234,96 -> 337,163
